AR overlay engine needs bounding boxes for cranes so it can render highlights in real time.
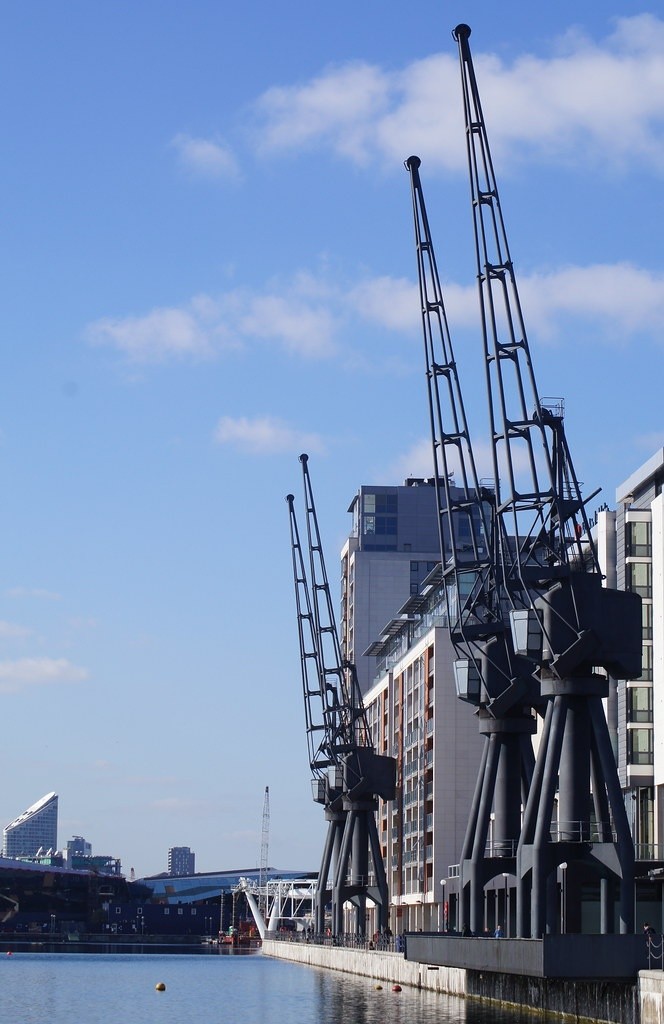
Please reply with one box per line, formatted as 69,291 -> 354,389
252,786 -> 271,920
284,452 -> 399,951
401,22 -> 649,972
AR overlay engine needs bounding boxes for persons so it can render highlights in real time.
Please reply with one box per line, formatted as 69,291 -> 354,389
461,923 -> 470,937
494,925 -> 504,937
642,922 -> 655,934
275,924 -> 422,953
